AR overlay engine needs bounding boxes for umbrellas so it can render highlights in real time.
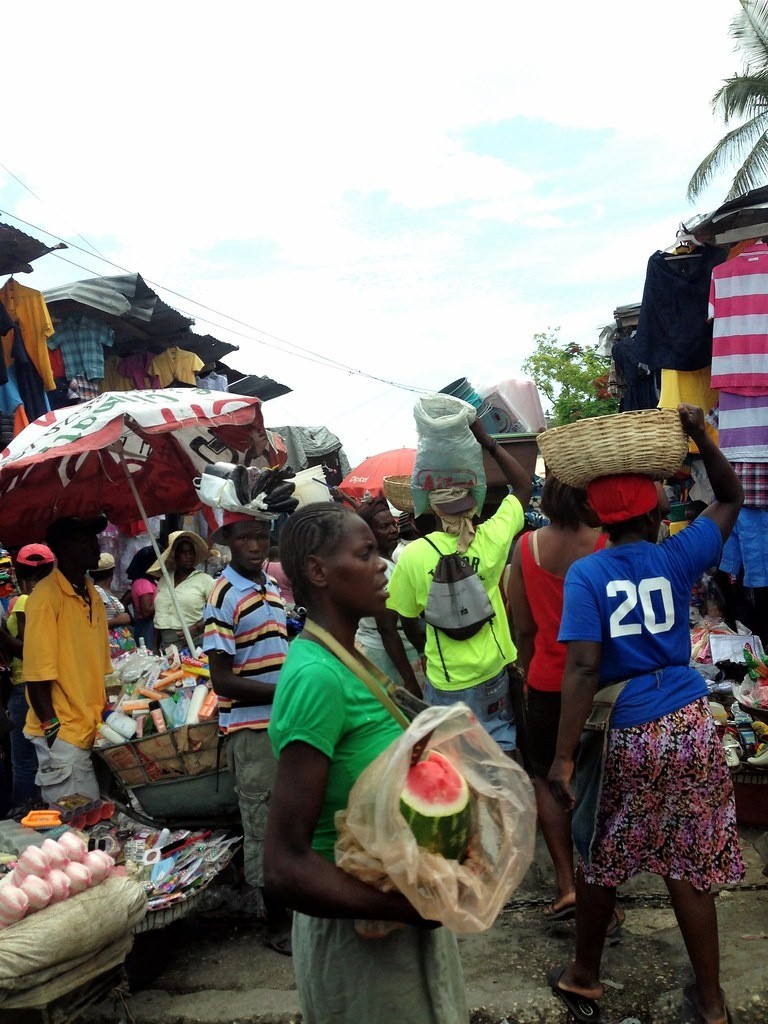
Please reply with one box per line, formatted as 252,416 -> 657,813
0,387 -> 287,659
340,448 -> 418,508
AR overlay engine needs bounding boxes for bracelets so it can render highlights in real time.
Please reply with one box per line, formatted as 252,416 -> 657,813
40,717 -> 60,738
489,438 -> 498,455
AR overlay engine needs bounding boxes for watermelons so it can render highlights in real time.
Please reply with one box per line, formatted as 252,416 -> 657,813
395,751 -> 470,867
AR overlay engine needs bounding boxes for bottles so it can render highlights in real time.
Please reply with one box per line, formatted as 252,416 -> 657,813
149,700 -> 166,735
737,722 -> 755,749
185,683 -> 208,725
101,710 -> 138,739
138,637 -> 147,657
96,723 -> 125,744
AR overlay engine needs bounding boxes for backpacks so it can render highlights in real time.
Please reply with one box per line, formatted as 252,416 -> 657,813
419,533 -> 496,642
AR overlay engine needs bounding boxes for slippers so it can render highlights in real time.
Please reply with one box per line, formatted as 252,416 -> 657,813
263,931 -> 293,956
605,905 -> 626,936
546,964 -> 601,1024
539,901 -> 576,921
683,978 -> 733,1024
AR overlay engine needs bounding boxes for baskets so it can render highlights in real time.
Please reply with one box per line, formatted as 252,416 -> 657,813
382,471 -> 436,515
536,407 -> 693,491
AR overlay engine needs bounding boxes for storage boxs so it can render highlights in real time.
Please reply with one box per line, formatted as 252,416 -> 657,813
198,689 -> 219,722
165,645 -> 181,671
157,696 -> 175,730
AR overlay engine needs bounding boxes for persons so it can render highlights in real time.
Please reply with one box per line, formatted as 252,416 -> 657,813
22,514 -> 114,806
89,552 -> 137,658
0,543 -> 55,802
262,546 -> 296,611
684,500 -> 708,525
146,531 -> 217,652
126,545 -> 166,649
355,496 -> 418,688
386,416 -> 533,761
545,402 -> 745,1024
506,482 -> 669,932
202,509 -> 292,955
262,499 -> 470,1024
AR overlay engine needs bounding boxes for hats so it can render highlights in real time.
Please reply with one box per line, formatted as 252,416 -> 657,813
88,552 -> 116,572
16,543 -> 55,567
46,515 -> 108,558
145,530 -> 209,578
210,510 -> 272,546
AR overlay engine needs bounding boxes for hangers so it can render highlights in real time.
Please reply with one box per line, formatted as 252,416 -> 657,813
209,369 -> 217,377
660,221 -> 706,260
